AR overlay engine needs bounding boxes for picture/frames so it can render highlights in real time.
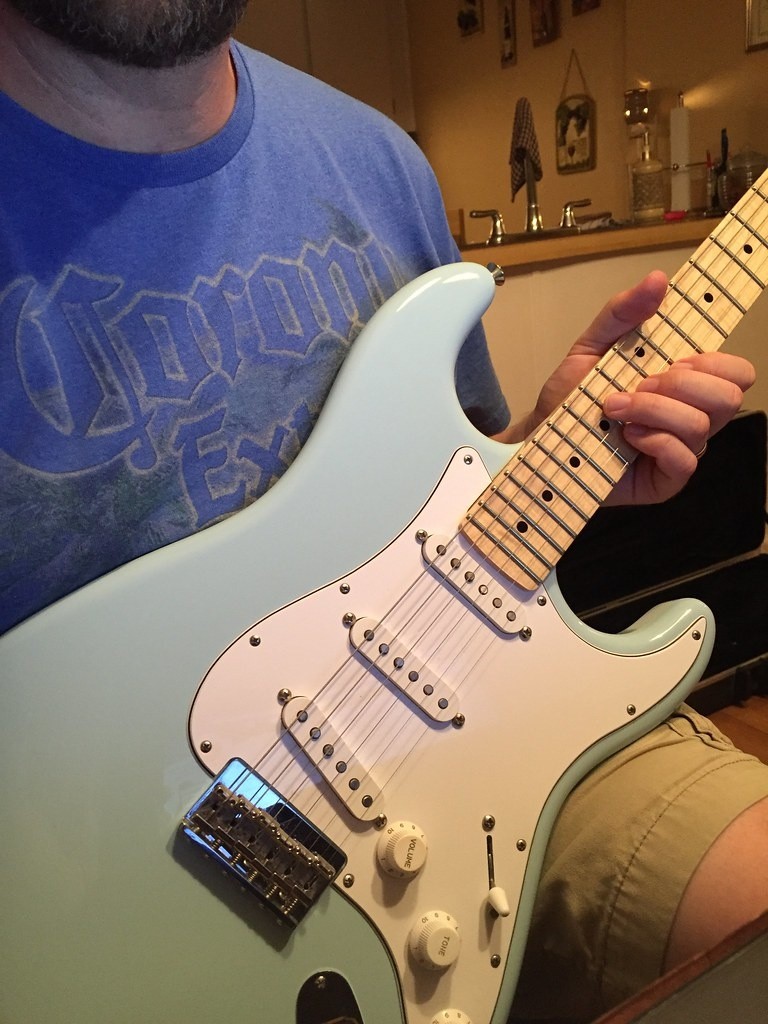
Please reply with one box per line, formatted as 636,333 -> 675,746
745,0 -> 768,54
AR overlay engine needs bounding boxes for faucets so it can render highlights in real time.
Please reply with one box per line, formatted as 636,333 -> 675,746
521,154 -> 545,233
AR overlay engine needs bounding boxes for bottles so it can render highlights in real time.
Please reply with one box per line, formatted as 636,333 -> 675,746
631,144 -> 664,220
718,143 -> 768,213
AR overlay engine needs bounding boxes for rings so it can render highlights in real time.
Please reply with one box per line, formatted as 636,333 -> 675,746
696,441 -> 707,458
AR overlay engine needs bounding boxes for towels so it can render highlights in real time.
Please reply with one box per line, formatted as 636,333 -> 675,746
508,96 -> 543,202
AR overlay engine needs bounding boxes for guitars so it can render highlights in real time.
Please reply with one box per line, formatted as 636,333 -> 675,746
2,164 -> 767,1024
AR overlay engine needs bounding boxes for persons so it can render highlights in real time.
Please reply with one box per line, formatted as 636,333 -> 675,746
0,0 -> 768,1024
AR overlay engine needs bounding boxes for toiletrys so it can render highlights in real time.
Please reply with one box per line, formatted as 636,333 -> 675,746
624,128 -> 667,224
